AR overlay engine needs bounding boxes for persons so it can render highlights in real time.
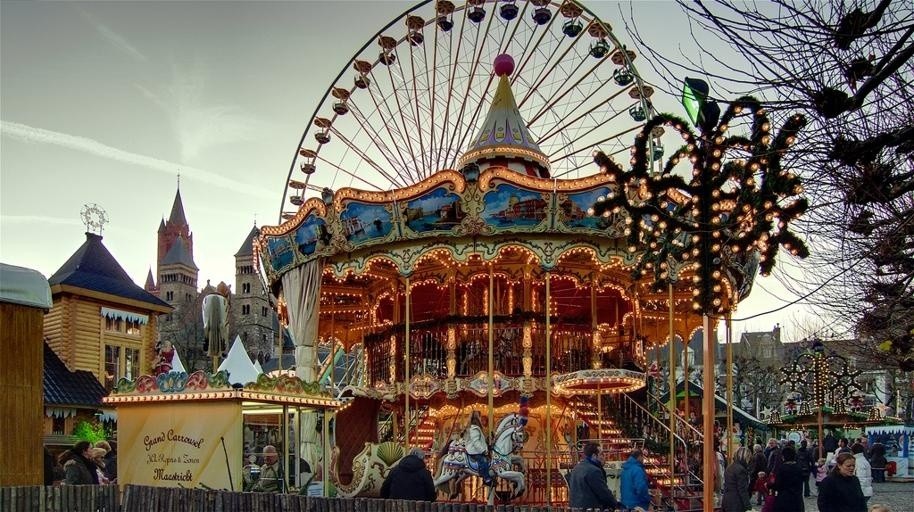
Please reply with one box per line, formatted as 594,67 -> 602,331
243,445 -> 312,493
460,411 -> 492,486
380,447 -> 437,502
43,440 -> 118,485
620,448 -> 651,511
568,443 -> 617,509
713,431 -> 897,512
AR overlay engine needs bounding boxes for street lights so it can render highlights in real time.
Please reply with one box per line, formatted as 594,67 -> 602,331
676,74 -> 722,511
811,340 -> 827,461
